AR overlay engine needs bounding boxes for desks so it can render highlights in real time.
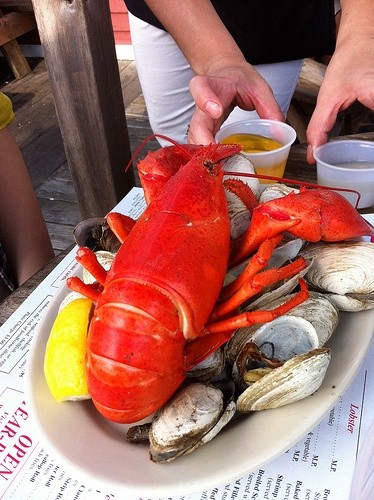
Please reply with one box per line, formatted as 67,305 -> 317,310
1,143 -> 374,500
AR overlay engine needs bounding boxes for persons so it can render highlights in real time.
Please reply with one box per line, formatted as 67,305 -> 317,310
0,91 -> 55,288
123,0 -> 374,166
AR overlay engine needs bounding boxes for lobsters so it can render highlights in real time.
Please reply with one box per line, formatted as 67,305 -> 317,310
66,135 -> 372,425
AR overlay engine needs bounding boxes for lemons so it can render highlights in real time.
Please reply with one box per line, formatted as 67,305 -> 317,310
43,299 -> 95,403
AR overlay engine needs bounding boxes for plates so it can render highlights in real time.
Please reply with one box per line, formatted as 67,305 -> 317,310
22,234 -> 374,496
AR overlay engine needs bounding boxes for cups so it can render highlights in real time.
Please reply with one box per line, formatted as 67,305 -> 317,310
312,139 -> 374,209
215,119 -> 297,184
